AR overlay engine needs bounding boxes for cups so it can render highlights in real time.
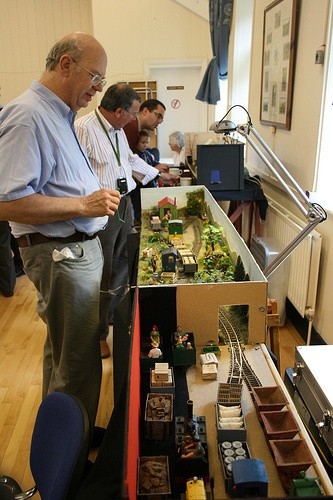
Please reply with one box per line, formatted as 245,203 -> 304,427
180,177 -> 192,186
169,168 -> 182,177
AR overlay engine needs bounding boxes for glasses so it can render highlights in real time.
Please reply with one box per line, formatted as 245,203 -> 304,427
152,111 -> 163,121
125,110 -> 136,119
70,57 -> 106,87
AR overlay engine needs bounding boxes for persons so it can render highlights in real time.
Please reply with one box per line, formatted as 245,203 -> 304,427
181,436 -> 205,458
76,83 -> 180,358
167,210 -> 171,219
174,326 -> 193,349
148,341 -> 162,358
0,34 -> 120,445
123,99 -> 166,220
150,325 -> 160,343
168,131 -> 184,167
134,130 -> 162,188
151,255 -> 158,272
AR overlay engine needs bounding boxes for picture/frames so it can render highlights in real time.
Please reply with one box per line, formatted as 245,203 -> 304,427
260,0 -> 300,130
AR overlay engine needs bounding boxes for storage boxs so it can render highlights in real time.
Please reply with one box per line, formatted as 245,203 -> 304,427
294,345 -> 333,455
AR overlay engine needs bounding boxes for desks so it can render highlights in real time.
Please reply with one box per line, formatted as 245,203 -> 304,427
211,178 -> 267,247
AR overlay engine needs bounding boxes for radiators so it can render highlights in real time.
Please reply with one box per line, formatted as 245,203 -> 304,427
251,192 -> 321,318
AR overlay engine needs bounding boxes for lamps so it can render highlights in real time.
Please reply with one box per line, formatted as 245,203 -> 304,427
209,105 -> 253,137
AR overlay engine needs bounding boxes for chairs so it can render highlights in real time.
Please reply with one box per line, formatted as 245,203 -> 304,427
0,391 -> 91,500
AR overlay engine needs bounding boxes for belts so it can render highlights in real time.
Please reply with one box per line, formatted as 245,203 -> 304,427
15,229 -> 96,247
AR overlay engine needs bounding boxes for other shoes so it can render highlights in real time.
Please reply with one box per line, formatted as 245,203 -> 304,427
15,271 -> 23,276
89,425 -> 105,446
99,341 -> 110,357
